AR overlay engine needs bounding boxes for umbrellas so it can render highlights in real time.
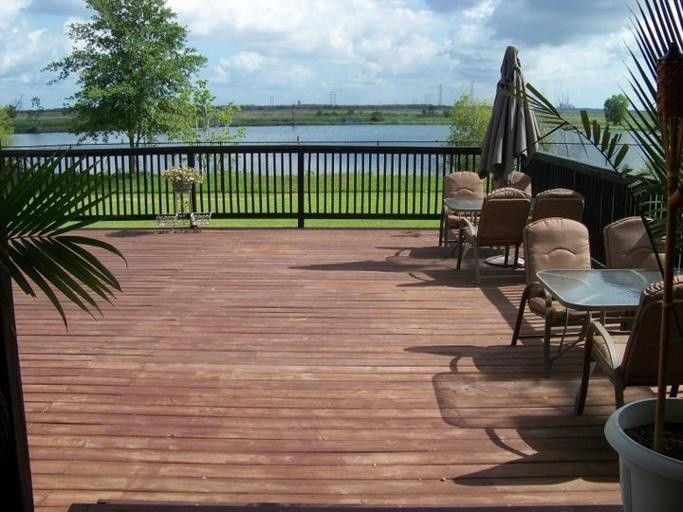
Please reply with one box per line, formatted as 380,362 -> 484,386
477,46 -> 541,180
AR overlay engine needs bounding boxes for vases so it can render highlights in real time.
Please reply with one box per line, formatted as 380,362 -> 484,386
170,180 -> 195,192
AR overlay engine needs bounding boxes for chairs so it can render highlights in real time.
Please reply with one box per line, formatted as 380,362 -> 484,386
601,216 -> 680,271
514,188 -> 585,269
494,172 -> 532,199
512,217 -> 598,376
575,276 -> 682,415
438,171 -> 484,248
458,187 -> 532,275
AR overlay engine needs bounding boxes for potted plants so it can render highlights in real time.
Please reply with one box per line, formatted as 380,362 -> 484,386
604,0 -> 681,512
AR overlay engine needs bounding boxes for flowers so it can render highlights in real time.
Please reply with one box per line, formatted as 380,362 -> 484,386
160,165 -> 205,185
